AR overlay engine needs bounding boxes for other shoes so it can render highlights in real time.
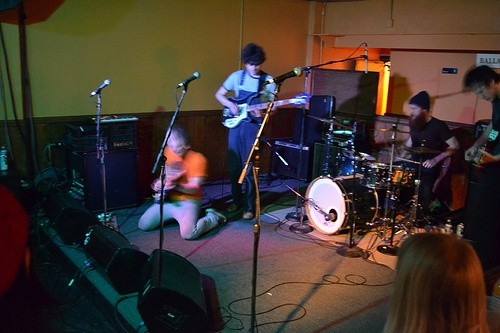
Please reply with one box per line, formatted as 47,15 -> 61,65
243,211 -> 254,219
226,203 -> 244,213
206,208 -> 226,224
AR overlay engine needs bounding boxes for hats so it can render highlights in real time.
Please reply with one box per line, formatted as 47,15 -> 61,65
410,91 -> 429,110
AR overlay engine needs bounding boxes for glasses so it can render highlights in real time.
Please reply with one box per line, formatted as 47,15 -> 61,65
247,62 -> 259,66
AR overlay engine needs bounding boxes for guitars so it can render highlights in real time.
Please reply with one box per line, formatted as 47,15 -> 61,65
221,93 -> 309,129
471,120 -> 493,168
153,170 -> 186,204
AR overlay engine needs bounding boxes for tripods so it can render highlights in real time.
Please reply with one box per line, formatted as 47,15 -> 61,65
359,124 -> 433,242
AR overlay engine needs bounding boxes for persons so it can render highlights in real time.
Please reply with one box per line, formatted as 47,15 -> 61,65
399,90 -> 459,218
464,65 -> 500,297
215,43 -> 276,220
384,232 -> 488,333
138,126 -> 227,240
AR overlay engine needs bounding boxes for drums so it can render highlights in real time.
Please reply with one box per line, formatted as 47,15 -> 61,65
392,168 -> 416,188
304,174 -> 379,235
360,163 -> 403,190
341,151 -> 377,176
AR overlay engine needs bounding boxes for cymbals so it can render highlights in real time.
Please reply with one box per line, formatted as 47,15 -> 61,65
376,128 -> 410,134
403,146 -> 441,154
306,115 -> 354,130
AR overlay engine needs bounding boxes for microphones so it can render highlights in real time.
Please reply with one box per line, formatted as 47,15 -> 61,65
263,67 -> 303,86
324,212 -> 336,222
176,71 -> 200,89
89,79 -> 111,97
364,44 -> 369,73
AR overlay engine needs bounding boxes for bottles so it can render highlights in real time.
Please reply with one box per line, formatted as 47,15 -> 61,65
0,145 -> 8,170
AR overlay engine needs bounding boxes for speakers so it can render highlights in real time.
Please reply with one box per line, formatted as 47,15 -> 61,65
28,166 -> 208,333
462,119 -> 493,257
311,68 -> 379,118
271,93 -> 348,182
